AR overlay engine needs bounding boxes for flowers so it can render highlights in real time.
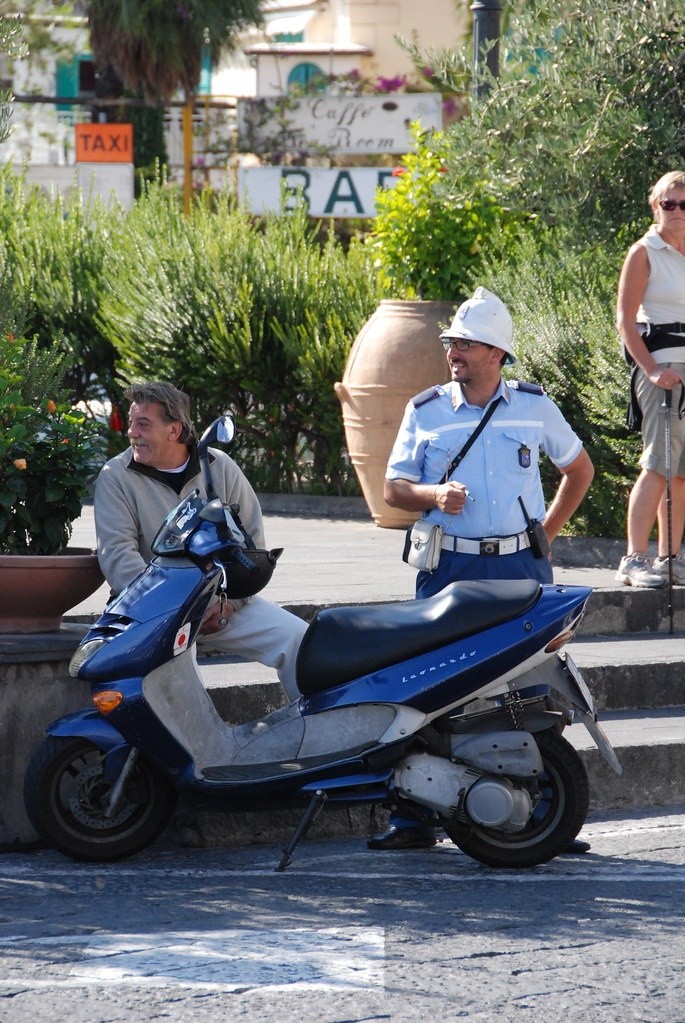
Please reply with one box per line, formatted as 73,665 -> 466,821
0,328 -> 110,555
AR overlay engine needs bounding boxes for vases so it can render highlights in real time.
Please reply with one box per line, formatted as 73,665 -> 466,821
332,115 -> 491,525
0,547 -> 106,634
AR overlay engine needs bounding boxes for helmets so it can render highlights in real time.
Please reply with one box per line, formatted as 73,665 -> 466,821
213,548 -> 284,600
438,286 -> 517,367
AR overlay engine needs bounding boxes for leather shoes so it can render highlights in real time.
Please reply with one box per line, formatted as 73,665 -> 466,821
367,824 -> 436,850
560,840 -> 590,853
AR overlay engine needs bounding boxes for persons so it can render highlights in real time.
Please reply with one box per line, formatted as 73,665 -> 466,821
93,381 -> 309,705
367,285 -> 596,856
615,168 -> 685,592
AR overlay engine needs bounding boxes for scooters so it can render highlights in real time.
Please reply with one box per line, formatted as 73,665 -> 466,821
21,417 -> 624,874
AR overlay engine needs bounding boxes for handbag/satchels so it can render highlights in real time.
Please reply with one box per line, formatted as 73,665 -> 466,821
623,324 -> 685,367
402,526 -> 412,563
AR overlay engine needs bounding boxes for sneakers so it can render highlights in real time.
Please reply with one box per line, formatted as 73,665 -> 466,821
614,551 -> 666,587
652,550 -> 685,585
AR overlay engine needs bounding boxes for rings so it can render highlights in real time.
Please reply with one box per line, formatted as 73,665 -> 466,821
671,381 -> 674,384
665,381 -> 669,384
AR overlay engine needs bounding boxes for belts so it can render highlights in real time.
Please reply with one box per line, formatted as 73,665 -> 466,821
438,530 -> 530,556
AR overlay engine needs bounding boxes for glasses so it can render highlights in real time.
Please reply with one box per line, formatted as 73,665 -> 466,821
656,201 -> 685,211
441,340 -> 483,351
